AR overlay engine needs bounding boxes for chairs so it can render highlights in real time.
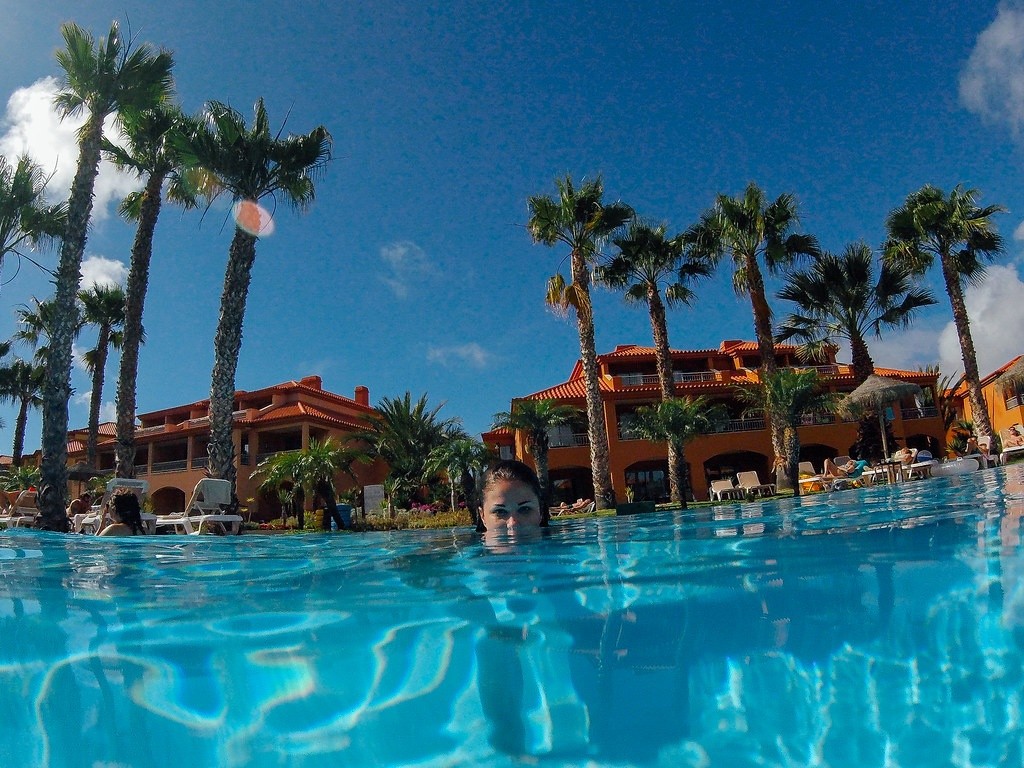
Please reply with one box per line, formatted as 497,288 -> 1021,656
0,490 -> 38,528
999,424 -> 1024,464
963,436 -> 999,469
709,479 -> 747,504
735,471 -> 776,499
82,477 -> 156,535
156,478 -> 243,536
798,449 -> 937,493
549,500 -> 595,517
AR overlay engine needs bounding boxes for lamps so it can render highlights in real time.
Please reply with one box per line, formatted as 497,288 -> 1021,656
495,445 -> 499,449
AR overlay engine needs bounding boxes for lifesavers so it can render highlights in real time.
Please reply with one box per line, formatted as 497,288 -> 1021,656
931,459 -> 979,477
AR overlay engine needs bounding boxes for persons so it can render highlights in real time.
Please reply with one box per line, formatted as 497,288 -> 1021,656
863,447 -> 913,471
66,492 -> 92,516
94,487 -> 133,536
473,460 -> 550,531
821,458 -> 857,478
1003,426 -> 1023,447
550,498 -> 591,512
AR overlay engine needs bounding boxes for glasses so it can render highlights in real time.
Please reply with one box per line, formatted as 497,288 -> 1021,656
84,499 -> 91,503
1010,428 -> 1015,431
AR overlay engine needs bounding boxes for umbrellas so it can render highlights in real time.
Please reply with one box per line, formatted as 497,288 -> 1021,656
838,374 -> 922,483
66,459 -> 107,499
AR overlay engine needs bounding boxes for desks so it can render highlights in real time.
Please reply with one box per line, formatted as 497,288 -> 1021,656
872,460 -> 904,485
